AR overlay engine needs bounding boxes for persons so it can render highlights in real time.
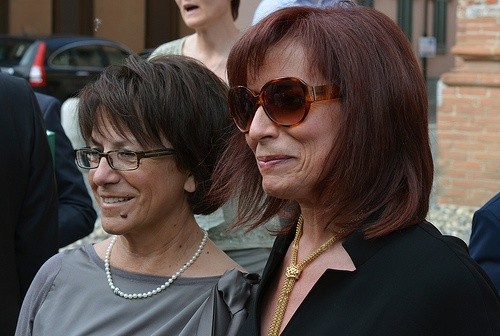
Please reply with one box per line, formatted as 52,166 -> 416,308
203,2 -> 500,336
0,71 -> 58,335
16,55 -> 261,336
35,90 -> 97,249
467,192 -> 500,293
146,0 -> 281,281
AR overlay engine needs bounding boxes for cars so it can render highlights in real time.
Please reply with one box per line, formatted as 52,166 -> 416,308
0,36 -> 142,102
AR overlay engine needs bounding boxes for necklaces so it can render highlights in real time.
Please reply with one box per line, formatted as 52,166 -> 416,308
104,225 -> 209,300
268,212 -> 361,336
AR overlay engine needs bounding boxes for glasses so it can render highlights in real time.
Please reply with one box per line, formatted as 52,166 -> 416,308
74,147 -> 177,170
229,77 -> 345,133
